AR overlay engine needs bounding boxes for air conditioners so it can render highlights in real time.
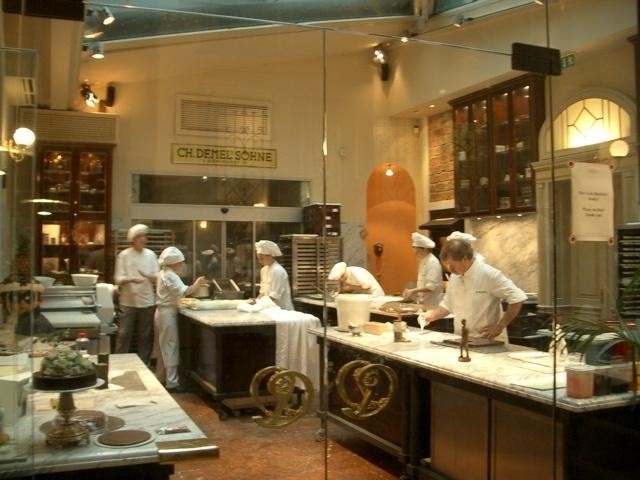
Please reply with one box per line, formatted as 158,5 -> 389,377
2,46 -> 40,108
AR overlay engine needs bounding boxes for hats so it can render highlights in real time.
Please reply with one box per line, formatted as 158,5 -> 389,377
254,239 -> 283,257
210,243 -> 219,252
175,243 -> 189,249
226,247 -> 235,254
446,231 -> 477,242
328,262 -> 347,280
411,232 -> 436,249
126,224 -> 150,243
201,249 -> 215,256
158,246 -> 186,267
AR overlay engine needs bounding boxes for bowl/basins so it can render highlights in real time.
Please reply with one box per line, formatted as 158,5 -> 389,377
34,277 -> 56,286
71,274 -> 99,287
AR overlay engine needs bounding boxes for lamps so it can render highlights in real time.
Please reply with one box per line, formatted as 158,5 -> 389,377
98,7 -> 113,29
82,10 -> 105,38
609,139 -> 632,160
79,79 -> 102,108
83,41 -> 105,59
453,14 -> 467,27
0,126 -> 37,162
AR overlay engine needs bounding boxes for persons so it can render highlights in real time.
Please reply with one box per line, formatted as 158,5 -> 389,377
328,262 -> 386,297
227,247 -> 244,280
417,239 -> 528,344
113,223 -> 160,370
152,247 -> 211,393
248,239 -> 295,311
448,230 -> 491,263
403,232 -> 443,312
201,249 -> 221,281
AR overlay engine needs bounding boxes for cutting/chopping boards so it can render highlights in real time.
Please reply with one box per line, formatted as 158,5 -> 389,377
445,336 -> 505,348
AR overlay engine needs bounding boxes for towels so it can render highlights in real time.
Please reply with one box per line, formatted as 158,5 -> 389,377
262,310 -> 321,393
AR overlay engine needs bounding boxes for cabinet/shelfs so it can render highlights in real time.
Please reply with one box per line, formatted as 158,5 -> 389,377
302,204 -> 342,235
110,228 -> 175,292
278,234 -> 343,298
17,144 -> 118,284
448,73 -> 546,218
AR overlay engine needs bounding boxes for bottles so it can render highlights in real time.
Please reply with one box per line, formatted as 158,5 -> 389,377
392,319 -> 402,342
75,332 -> 91,354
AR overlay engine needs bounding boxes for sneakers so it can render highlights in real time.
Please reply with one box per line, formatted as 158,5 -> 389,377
167,383 -> 189,393
